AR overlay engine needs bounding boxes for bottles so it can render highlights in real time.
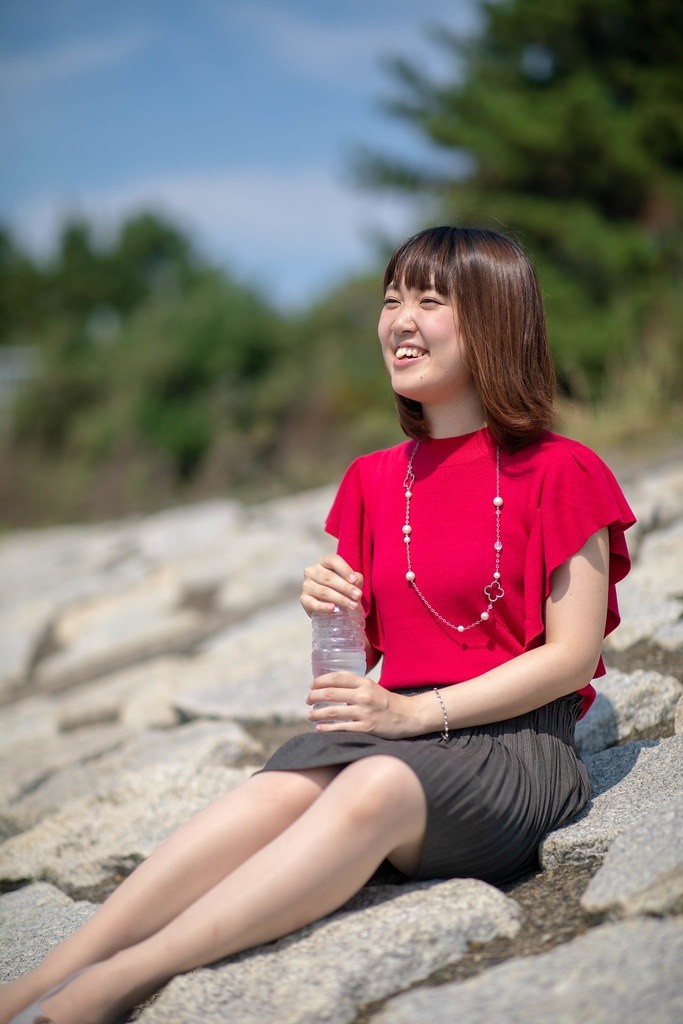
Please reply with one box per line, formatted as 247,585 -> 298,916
309,571 -> 368,731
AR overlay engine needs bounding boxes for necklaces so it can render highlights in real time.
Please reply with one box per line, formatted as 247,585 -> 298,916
402,439 -> 503,632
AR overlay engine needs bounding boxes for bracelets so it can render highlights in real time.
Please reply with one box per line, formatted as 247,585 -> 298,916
434,688 -> 449,742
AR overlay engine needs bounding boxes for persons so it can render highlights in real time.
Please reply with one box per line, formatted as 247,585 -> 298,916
0,227 -> 637,1024
